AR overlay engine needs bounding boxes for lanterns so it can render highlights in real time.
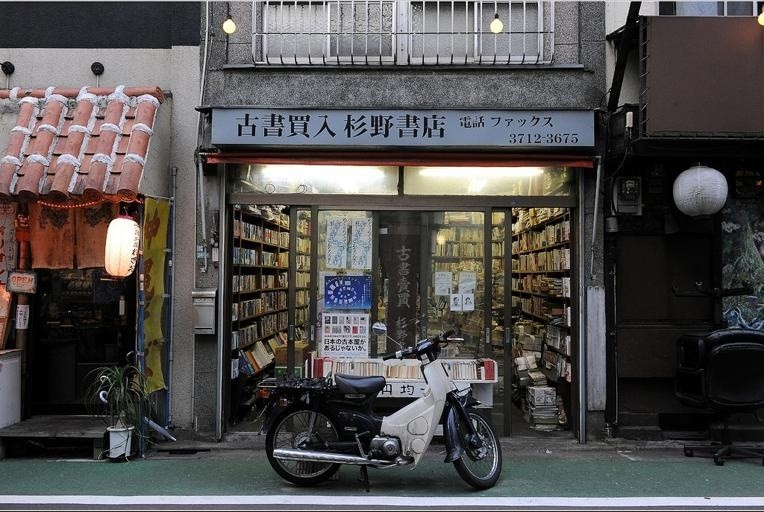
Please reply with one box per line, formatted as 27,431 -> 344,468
673,164 -> 728,221
103,214 -> 140,282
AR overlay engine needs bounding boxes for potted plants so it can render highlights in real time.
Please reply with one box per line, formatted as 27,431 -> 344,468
82,364 -> 155,459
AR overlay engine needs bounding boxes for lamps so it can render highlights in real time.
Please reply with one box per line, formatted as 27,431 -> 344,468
222,2 -> 236,35
488,2 -> 504,35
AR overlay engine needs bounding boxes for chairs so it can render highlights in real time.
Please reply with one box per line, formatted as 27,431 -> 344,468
673,329 -> 762,464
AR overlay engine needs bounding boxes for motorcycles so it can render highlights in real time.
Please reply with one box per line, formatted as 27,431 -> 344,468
249,320 -> 503,492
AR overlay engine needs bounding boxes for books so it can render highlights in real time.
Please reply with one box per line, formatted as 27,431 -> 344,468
229,203 -> 327,382
512,169 -> 571,197
429,205 -> 506,399
327,357 -> 422,381
511,207 -> 574,434
